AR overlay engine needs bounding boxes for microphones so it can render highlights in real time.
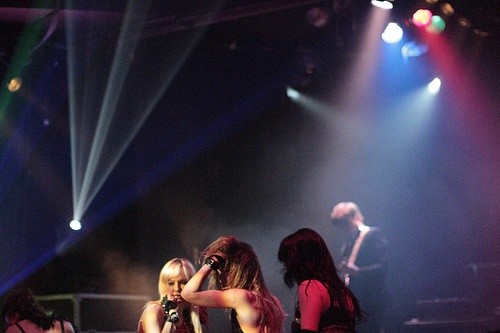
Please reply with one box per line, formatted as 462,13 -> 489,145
168,302 -> 178,323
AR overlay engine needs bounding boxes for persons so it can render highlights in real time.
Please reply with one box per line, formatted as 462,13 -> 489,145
181,236 -> 288,333
331,201 -> 389,333
137,258 -> 208,333
0,285 -> 75,333
278,228 -> 362,333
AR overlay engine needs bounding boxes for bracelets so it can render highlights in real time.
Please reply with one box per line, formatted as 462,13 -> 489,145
164,316 -> 172,321
205,254 -> 224,272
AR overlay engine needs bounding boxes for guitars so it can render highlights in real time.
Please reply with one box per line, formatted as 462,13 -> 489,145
334,255 -> 350,285
